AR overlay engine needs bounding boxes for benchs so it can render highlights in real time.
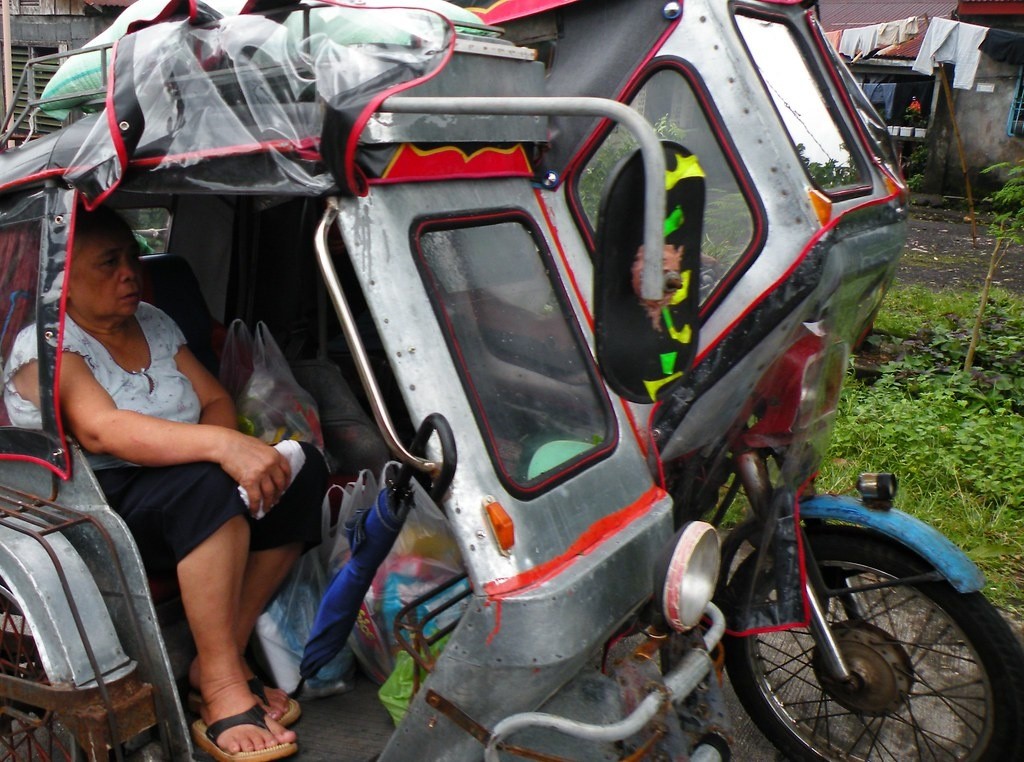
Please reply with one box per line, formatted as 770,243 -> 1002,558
140,252 -> 365,627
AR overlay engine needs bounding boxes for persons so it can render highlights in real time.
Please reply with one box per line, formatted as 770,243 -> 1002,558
2,203 -> 331,762
910,96 -> 920,112
425,234 -> 602,424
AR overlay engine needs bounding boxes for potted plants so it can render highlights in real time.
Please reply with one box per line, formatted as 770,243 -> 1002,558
879,106 -> 929,138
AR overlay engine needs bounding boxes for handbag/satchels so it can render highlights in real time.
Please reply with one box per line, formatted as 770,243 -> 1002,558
215,320 -> 325,467
253,462 -> 472,730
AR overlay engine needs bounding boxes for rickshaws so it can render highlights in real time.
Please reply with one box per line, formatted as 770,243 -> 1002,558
0,0 -> 1024,761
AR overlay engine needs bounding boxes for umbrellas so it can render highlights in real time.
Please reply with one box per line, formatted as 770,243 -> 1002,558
292,413 -> 459,700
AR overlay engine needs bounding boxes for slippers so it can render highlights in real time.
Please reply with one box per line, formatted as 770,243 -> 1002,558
191,704 -> 298,762
186,679 -> 301,728
